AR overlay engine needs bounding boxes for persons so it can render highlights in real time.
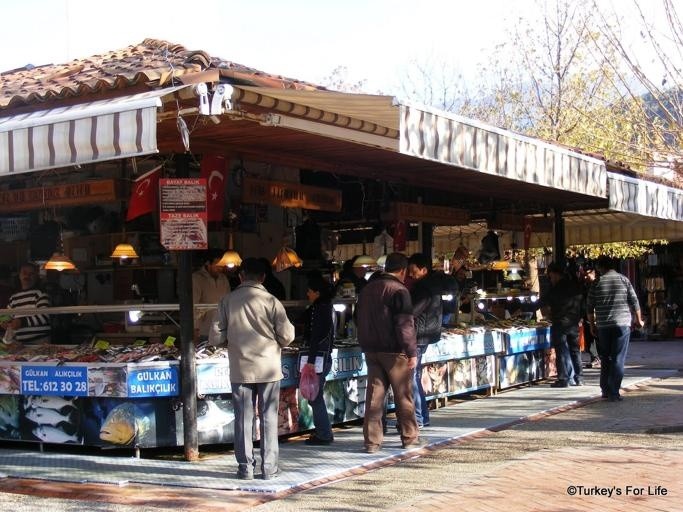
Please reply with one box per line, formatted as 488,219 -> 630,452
303,270 -> 334,445
353,252 -> 428,452
191,248 -> 232,304
209,258 -> 295,478
0,263 -> 53,346
407,253 -> 442,428
520,262 -> 582,387
586,256 -> 644,401
585,265 -> 601,368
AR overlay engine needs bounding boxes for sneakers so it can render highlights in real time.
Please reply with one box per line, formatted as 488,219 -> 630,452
402,437 -> 430,450
367,443 -> 382,454
550,382 -> 570,388
608,395 -> 624,402
305,435 -> 335,446
418,419 -> 431,430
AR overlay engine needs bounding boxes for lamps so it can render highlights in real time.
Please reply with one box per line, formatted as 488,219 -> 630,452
504,208 -> 523,281
213,157 -> 244,269
270,163 -> 303,273
352,176 -> 377,270
376,238 -> 389,269
108,177 -> 141,260
40,176 -> 76,272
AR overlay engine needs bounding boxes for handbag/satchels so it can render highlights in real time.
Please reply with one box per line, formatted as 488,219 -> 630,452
295,348 -> 328,375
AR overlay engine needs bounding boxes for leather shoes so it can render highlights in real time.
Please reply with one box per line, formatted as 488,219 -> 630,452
236,470 -> 253,480
262,469 -> 283,480
